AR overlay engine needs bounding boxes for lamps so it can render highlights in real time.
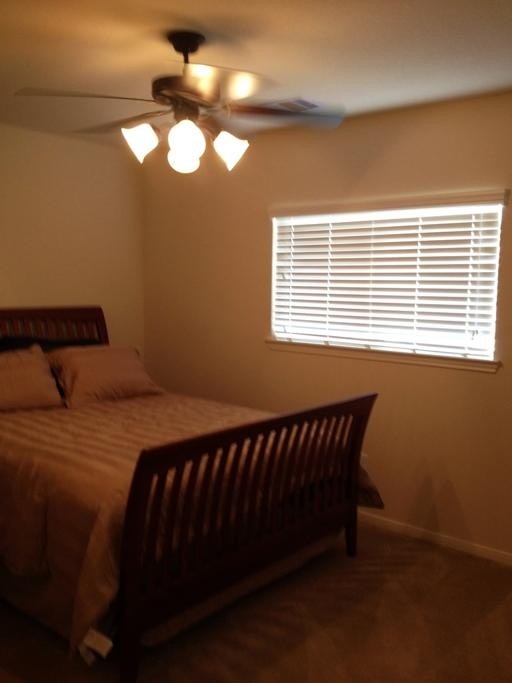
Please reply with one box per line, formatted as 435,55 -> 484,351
120,105 -> 250,175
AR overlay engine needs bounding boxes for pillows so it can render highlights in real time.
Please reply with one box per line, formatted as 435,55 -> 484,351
0,343 -> 166,410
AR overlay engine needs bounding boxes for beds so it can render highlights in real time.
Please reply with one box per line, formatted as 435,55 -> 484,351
0,305 -> 378,681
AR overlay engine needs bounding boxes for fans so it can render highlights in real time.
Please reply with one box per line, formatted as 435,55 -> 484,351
32,29 -> 346,137
